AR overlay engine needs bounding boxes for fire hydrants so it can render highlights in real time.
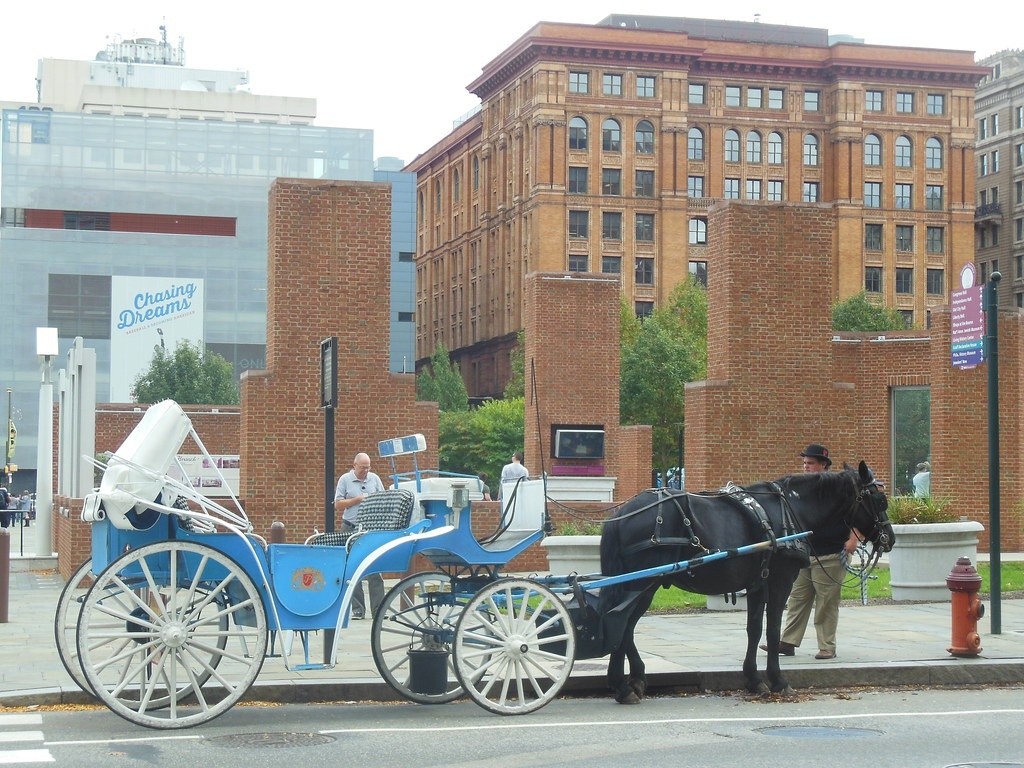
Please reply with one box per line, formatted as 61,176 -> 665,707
945,554 -> 987,659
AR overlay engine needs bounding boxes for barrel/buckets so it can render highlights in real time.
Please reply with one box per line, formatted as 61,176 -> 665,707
407,617 -> 450,694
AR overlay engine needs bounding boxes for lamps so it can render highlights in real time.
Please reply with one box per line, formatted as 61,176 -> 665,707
447,481 -> 469,530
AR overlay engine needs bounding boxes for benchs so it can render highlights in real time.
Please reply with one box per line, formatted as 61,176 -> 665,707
171,497 -> 195,532
303,434 -> 485,549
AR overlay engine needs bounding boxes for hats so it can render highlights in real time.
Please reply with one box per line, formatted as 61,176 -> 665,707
799,445 -> 832,469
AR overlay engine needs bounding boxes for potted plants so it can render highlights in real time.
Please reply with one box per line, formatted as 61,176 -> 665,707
885,489 -> 984,601
540,518 -> 604,604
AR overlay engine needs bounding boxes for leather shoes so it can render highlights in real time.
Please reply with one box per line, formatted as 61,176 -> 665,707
814,648 -> 836,659
759,641 -> 795,656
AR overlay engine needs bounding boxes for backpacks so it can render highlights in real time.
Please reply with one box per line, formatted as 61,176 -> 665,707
0,491 -> 7,509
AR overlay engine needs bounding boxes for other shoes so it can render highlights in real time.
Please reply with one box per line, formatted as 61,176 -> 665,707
373,613 -> 389,620
351,611 -> 365,620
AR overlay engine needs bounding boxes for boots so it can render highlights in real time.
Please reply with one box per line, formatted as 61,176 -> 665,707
24,518 -> 29,527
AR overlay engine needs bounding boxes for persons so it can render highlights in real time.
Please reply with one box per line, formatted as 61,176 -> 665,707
759,445 -> 861,659
475,472 -> 493,501
497,451 -> 529,501
0,483 -> 30,527
333,453 -> 390,620
913,463 -> 929,497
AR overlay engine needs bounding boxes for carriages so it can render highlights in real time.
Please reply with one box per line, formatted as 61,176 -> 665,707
55,401 -> 897,729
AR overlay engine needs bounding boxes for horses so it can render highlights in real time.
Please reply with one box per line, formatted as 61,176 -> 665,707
600,460 -> 895,704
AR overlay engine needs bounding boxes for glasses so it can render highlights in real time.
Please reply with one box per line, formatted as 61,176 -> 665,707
354,464 -> 372,471
